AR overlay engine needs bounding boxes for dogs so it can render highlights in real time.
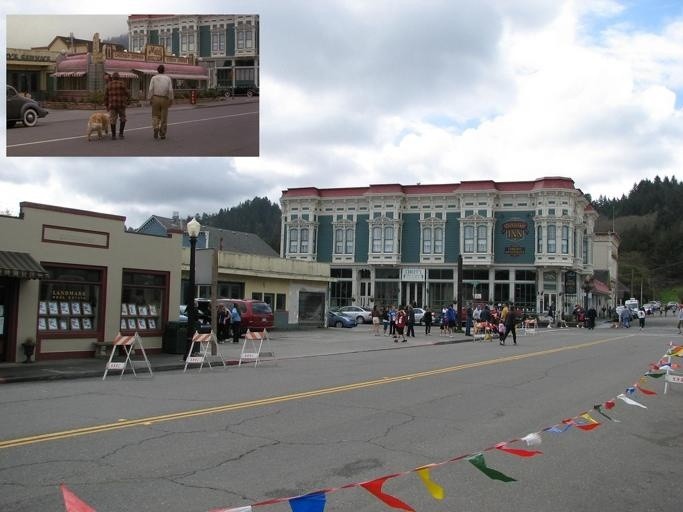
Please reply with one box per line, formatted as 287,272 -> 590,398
86,112 -> 112,142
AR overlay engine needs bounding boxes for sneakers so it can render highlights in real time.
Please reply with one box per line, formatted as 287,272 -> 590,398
154,128 -> 159,139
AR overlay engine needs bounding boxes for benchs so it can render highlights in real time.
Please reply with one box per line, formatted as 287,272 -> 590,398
90,340 -> 136,356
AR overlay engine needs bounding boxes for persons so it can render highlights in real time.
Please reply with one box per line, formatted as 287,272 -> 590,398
547,306 -> 554,328
572,302 -> 683,334
104,72 -> 131,139
195,300 -> 242,343
371,301 -> 522,346
147,65 -> 174,139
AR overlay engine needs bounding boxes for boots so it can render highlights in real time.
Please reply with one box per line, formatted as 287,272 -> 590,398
111,124 -> 116,140
119,122 -> 126,137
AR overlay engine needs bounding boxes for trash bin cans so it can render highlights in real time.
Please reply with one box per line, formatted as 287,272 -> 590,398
162,321 -> 200,354
191,89 -> 197,104
585,318 -> 589,327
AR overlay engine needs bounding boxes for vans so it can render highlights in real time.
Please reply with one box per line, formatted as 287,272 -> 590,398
217,298 -> 273,335
220,79 -> 258,99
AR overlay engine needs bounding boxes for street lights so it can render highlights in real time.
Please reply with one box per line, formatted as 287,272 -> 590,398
580,276 -> 592,310
185,216 -> 199,360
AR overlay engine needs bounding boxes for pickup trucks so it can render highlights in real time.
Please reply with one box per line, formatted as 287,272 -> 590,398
461,301 -> 523,325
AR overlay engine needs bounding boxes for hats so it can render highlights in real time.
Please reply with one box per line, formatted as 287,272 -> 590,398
111,73 -> 120,78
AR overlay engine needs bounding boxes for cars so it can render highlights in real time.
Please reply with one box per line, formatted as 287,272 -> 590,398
329,309 -> 356,331
340,305 -> 371,323
6,84 -> 48,129
407,307 -> 436,327
179,298 -> 210,333
624,297 -> 682,319
